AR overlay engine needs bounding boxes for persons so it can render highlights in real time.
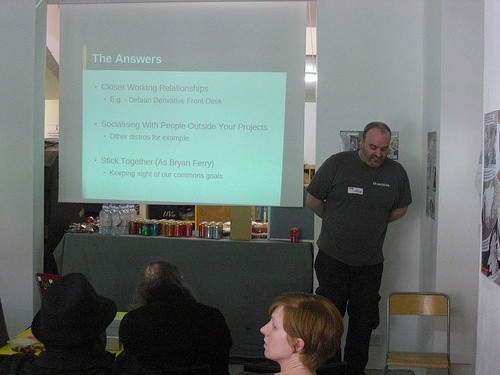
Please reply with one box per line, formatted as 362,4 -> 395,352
119,257 -> 235,375
1,272 -> 145,375
305,119 -> 414,375
258,290 -> 344,375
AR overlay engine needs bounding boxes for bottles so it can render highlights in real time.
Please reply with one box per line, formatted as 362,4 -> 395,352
99,203 -> 138,235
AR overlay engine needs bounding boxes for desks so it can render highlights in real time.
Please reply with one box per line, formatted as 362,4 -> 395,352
58,228 -> 314,346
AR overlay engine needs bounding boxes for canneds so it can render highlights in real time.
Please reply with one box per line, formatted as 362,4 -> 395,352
185,222 -> 192,237
128,217 -> 185,237
199,222 -> 224,239
290,228 -> 300,243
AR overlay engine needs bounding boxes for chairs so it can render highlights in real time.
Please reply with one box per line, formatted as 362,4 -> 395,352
383,292 -> 451,375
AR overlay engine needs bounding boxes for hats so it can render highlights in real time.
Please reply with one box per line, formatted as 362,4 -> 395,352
32,271 -> 117,345
